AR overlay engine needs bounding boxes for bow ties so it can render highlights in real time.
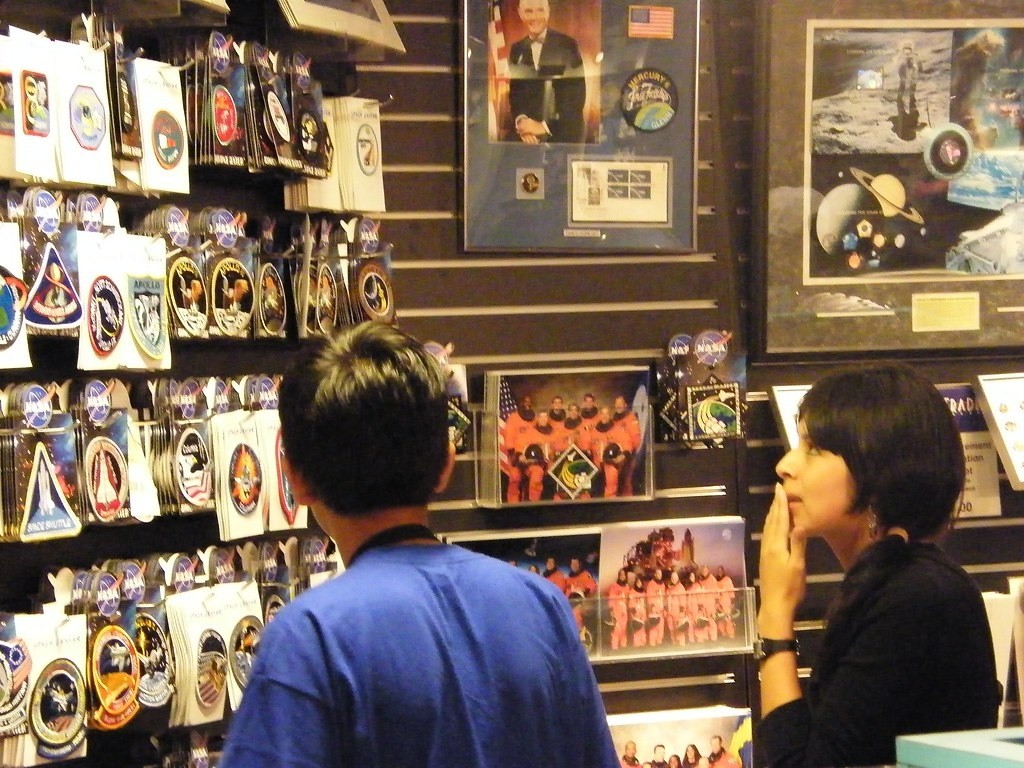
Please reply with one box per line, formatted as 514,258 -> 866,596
527,36 -> 545,43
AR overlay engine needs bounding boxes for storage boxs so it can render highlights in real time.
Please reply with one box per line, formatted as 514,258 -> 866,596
473,402 -> 653,510
568,585 -> 759,665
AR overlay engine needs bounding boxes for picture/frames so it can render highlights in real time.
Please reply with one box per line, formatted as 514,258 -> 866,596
751,0 -> 1024,372
456,0 -> 701,254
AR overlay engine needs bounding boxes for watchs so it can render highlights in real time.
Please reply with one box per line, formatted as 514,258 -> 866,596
753,637 -> 800,659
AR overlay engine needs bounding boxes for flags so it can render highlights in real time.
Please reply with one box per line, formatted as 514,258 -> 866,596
498,377 -> 518,477
488,0 -> 510,129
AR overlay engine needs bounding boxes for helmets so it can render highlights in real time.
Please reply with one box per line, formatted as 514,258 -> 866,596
524,444 -> 543,461
648,616 -> 660,627
569,590 -> 586,604
713,610 -> 726,620
732,609 -> 740,619
629,618 -> 644,631
603,443 -> 621,462
696,617 -> 709,627
678,619 -> 689,632
604,617 -> 616,628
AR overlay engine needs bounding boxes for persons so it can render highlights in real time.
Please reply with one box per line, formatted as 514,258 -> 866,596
609,568 -> 736,650
621,735 -> 744,768
502,393 -> 643,502
508,554 -> 597,642
215,323 -> 622,768
503,0 -> 584,142
749,362 -> 1004,768
